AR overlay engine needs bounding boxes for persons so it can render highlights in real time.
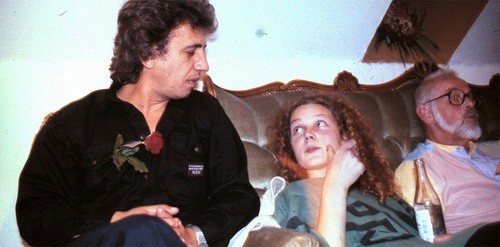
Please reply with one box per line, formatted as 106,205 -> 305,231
394,69 -> 500,234
273,94 -> 500,247
16,0 -> 260,247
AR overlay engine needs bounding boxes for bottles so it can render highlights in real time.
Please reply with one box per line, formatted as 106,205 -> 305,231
413,158 -> 447,243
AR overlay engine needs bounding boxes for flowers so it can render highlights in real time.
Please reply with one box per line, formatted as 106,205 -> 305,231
112,130 -> 164,173
374,1 -> 441,65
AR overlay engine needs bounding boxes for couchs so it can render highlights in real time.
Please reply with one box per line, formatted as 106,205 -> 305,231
203,60 -> 500,247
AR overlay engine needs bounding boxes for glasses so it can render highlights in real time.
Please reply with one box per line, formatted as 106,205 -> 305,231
423,88 -> 481,106
